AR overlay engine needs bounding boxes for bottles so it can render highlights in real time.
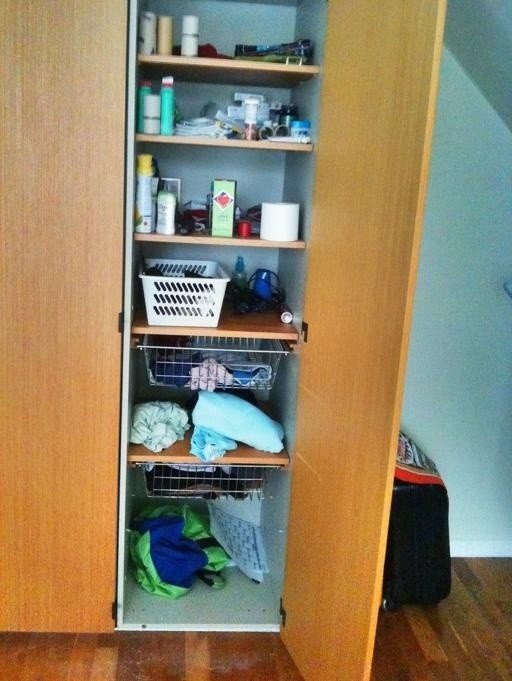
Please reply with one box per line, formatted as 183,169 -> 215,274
182,15 -> 198,58
138,9 -> 156,55
143,94 -> 162,134
156,14 -> 173,55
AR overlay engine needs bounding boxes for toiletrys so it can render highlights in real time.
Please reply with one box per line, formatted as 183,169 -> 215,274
291,120 -> 310,137
280,105 -> 298,136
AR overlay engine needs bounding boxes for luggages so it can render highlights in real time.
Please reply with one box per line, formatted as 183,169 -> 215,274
382,482 -> 452,612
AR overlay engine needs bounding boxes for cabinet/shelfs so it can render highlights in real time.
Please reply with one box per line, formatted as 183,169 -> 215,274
0,2 -> 446,681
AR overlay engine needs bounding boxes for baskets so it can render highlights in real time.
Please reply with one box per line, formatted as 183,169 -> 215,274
135,335 -> 290,389
133,463 -> 282,500
136,256 -> 232,329
